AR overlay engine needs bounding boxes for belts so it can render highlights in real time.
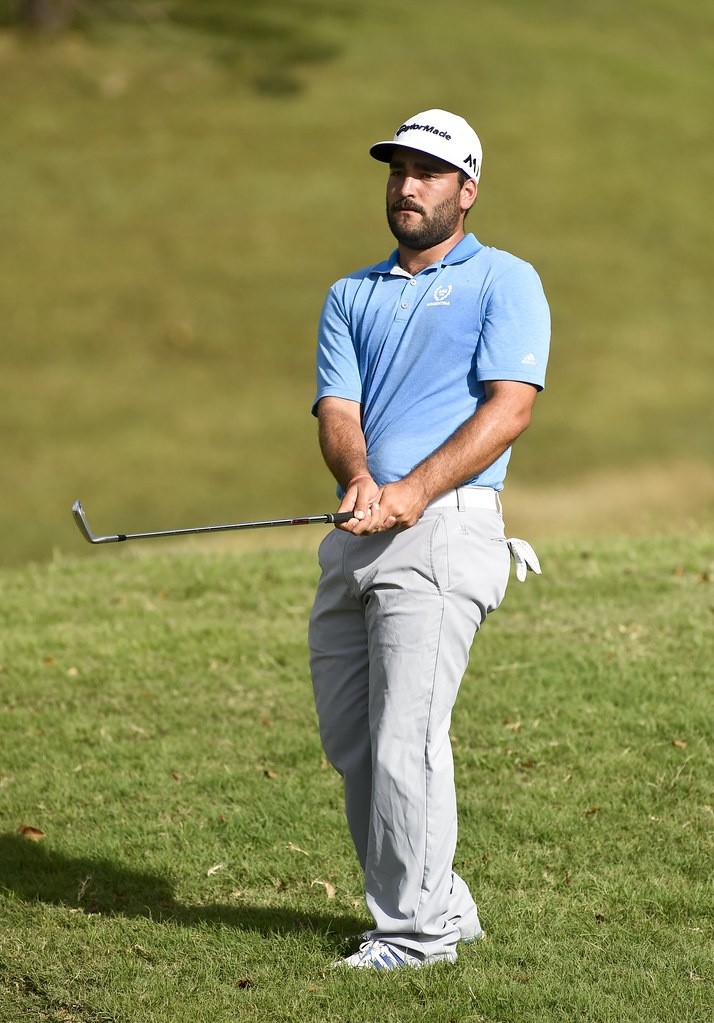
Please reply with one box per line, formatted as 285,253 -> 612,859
422,484 -> 500,510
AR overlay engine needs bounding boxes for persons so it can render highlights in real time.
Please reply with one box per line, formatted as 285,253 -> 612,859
307,108 -> 553,970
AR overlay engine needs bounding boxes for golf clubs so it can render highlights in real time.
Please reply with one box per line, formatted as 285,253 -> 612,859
70,499 -> 424,545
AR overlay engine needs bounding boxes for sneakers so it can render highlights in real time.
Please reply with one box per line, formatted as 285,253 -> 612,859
355,929 -> 485,943
331,938 -> 455,969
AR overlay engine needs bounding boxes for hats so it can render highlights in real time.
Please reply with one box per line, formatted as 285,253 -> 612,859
369,108 -> 483,184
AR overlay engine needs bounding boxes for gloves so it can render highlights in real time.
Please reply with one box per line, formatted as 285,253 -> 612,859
505,538 -> 542,582
